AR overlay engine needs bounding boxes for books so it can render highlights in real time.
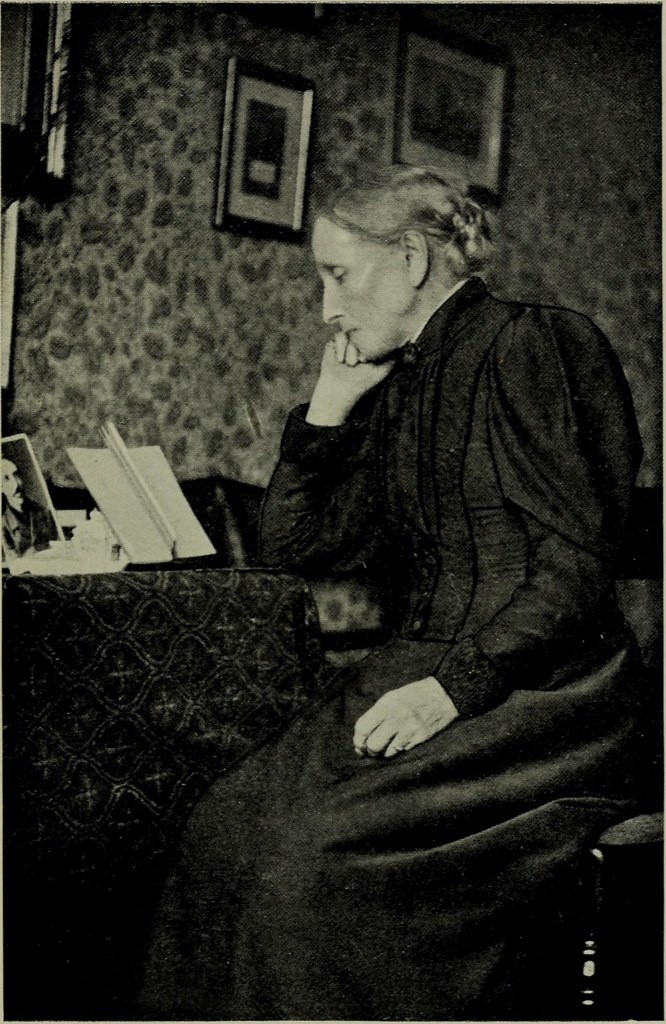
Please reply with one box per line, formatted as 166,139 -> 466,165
67,421 -> 216,563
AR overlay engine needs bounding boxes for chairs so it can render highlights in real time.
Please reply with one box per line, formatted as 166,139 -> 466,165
566,486 -> 664,1022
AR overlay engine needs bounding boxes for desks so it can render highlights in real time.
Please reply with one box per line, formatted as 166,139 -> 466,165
2,569 -> 326,1023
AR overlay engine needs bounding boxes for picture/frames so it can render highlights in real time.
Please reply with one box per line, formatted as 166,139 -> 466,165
1,3 -> 81,202
387,18 -> 513,208
212,54 -> 318,242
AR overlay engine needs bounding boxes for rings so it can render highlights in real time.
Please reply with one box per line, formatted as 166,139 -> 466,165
390,740 -> 404,750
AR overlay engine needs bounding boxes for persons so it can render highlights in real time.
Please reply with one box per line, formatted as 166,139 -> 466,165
2,458 -> 59,557
139,165 -> 643,1021
